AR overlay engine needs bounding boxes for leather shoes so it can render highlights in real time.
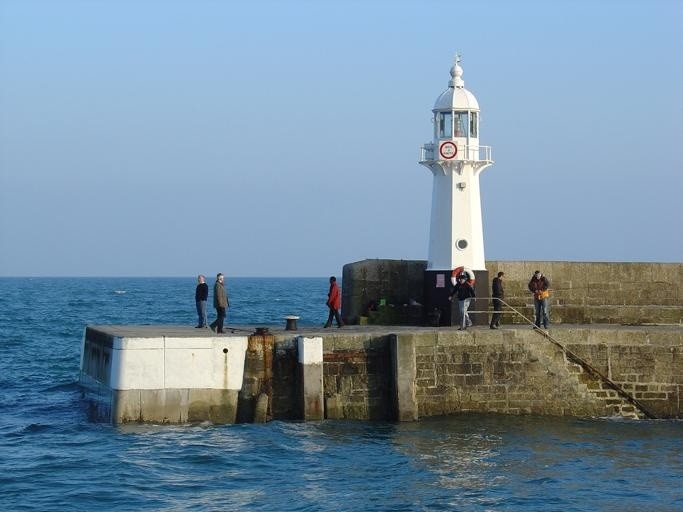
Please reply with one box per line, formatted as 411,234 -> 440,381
210,324 -> 216,333
217,330 -> 226,333
195,325 -> 204,328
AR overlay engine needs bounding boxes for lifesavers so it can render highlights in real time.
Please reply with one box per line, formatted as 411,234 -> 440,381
451,266 -> 476,286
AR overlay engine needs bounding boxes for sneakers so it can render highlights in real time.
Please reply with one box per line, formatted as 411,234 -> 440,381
323,324 -> 333,328
458,328 -> 466,330
544,326 -> 549,329
533,325 -> 540,329
490,325 -> 497,329
337,323 -> 345,328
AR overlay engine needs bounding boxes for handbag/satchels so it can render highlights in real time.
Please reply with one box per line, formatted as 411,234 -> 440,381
537,291 -> 549,300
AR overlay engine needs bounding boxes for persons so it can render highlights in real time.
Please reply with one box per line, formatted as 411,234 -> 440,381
490,272 -> 505,328
528,271 -> 550,329
323,276 -> 344,328
448,275 -> 477,330
195,273 -> 208,328
210,273 -> 229,334
